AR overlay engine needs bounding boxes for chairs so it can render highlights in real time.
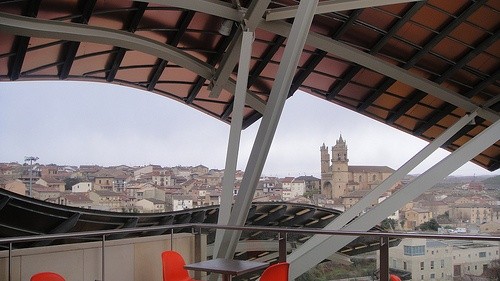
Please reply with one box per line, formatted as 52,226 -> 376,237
28,272 -> 65,281
259,261 -> 290,281
161,250 -> 199,281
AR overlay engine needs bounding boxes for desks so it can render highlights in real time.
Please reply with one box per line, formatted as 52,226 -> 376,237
183,257 -> 269,281
